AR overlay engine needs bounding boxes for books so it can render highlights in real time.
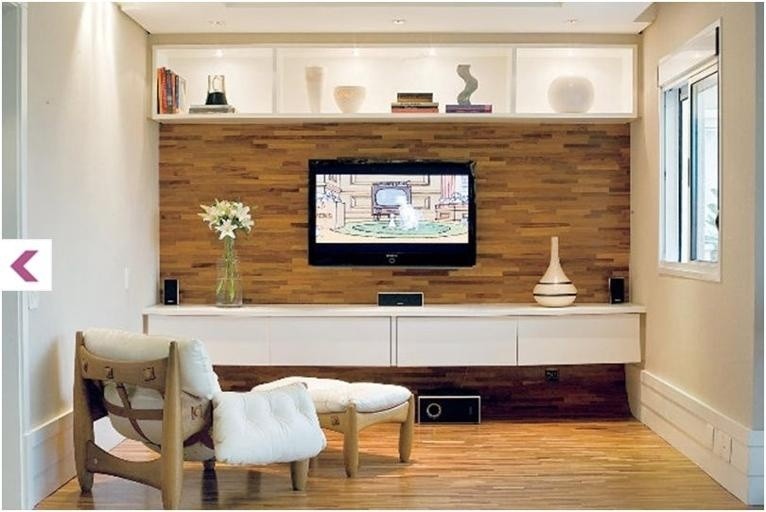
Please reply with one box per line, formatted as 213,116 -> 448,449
157,66 -> 188,115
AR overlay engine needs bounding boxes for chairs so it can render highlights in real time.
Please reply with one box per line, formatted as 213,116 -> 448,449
74,331 -> 312,509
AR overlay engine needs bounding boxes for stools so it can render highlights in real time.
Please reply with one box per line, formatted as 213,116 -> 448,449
251,376 -> 416,479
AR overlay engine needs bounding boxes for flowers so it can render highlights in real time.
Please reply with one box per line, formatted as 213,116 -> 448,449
197,199 -> 255,306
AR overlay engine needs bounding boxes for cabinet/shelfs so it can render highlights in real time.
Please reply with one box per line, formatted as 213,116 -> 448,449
140,304 -> 393,367
395,302 -> 645,367
145,32 -> 642,126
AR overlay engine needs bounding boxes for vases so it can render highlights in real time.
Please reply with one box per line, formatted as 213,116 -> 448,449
532,236 -> 579,307
547,78 -> 596,113
214,258 -> 245,307
334,86 -> 365,114
305,67 -> 324,113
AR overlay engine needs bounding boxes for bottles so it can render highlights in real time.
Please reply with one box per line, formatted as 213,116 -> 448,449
205,74 -> 227,104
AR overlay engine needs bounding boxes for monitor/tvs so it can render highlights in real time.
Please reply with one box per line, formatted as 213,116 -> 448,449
307,158 -> 476,271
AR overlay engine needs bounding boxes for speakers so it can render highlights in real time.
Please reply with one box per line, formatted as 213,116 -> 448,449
377,292 -> 425,307
418,395 -> 481,424
608,277 -> 624,303
163,278 -> 180,305
